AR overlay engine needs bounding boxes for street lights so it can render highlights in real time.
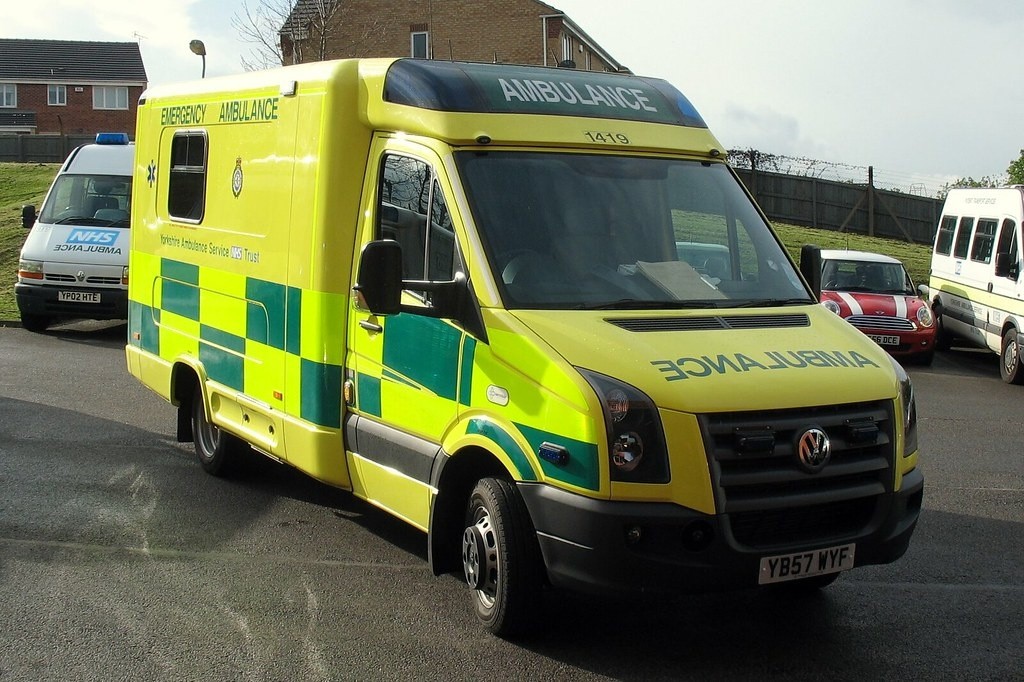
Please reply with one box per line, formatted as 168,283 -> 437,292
188,39 -> 207,78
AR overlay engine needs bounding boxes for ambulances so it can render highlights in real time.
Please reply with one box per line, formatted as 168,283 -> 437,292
927,187 -> 1024,386
124,57 -> 925,640
15,133 -> 135,331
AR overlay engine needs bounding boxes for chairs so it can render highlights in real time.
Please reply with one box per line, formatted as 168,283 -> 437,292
550,171 -> 647,275
83,181 -> 119,217
865,266 -> 885,287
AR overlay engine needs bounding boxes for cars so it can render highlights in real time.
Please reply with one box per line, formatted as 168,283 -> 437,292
821,249 -> 937,368
